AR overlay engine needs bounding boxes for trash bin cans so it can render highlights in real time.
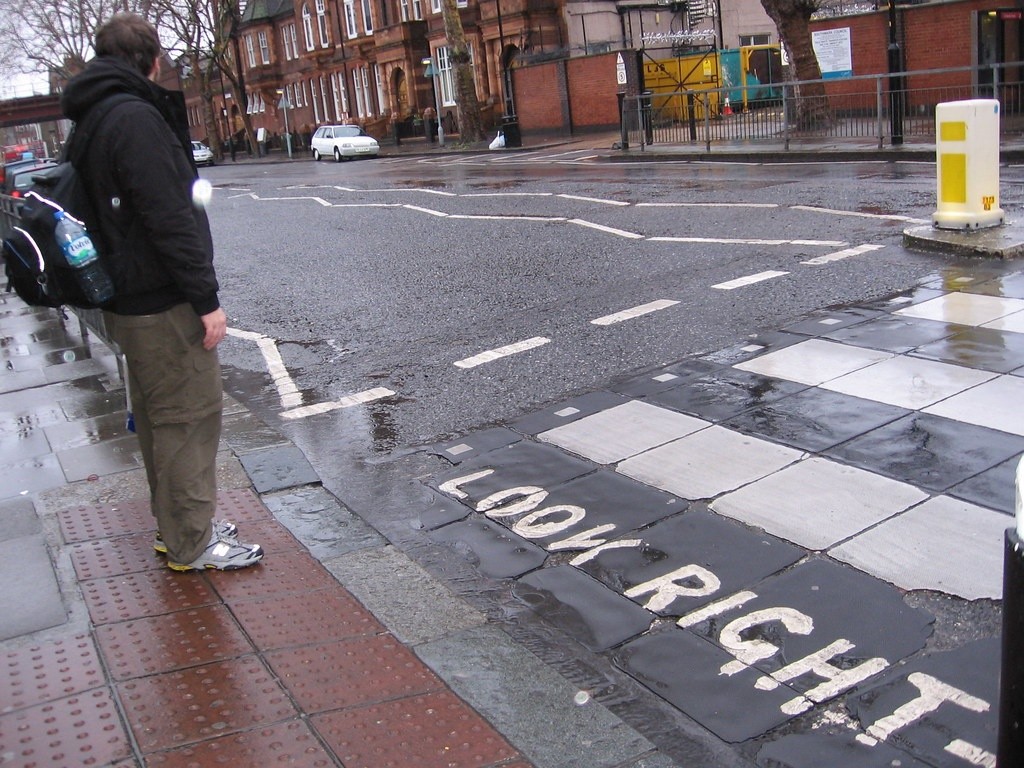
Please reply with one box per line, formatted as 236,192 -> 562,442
502,115 -> 523,148
931,99 -> 1004,230
280,132 -> 293,152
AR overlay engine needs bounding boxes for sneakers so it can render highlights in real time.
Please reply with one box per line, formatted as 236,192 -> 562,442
167,524 -> 264,571
153,517 -> 239,552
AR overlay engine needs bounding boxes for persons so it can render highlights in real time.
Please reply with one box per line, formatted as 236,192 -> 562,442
57,13 -> 265,573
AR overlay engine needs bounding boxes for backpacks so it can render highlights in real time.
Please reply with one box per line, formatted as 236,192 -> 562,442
2,93 -> 148,308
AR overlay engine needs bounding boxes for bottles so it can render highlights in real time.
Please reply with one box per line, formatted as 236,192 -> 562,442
54,210 -> 114,304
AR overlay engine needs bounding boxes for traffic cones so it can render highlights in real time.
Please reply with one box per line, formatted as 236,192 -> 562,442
720,92 -> 734,117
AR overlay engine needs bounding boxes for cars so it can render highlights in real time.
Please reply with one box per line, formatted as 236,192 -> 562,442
191,140 -> 214,166
0,139 -> 60,217
311,124 -> 381,163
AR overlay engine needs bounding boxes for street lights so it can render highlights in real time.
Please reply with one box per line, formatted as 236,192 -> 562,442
275,87 -> 293,159
421,57 -> 445,147
